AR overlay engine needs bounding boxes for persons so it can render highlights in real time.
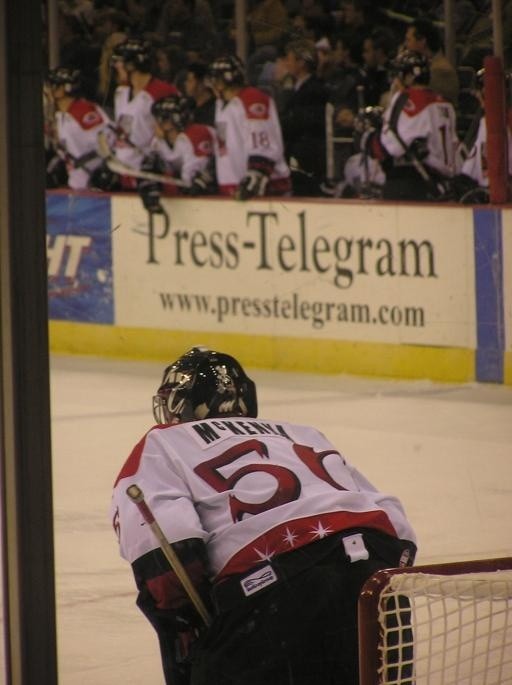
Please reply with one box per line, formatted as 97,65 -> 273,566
355,41 -> 455,204
146,93 -> 215,198
356,25 -> 395,105
111,345 -> 422,685
154,42 -> 182,88
453,66 -> 511,204
46,64 -> 116,191
92,8 -> 138,108
202,50 -> 298,198
38,1 -> 510,70
108,40 -> 184,196
279,36 -> 330,197
181,62 -> 216,126
404,17 -> 461,106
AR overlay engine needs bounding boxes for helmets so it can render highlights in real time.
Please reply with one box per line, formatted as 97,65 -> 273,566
43,62 -> 82,97
150,93 -> 196,133
385,45 -> 432,85
106,36 -> 158,75
202,52 -> 249,91
150,344 -> 258,426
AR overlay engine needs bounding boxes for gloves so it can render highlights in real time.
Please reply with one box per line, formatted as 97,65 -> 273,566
349,104 -> 382,156
238,154 -> 276,203
181,168 -> 211,197
88,165 -> 119,191
139,182 -> 164,214
45,150 -> 70,190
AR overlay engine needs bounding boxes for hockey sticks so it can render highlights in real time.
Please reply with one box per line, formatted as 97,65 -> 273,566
386,89 -> 445,194
95,33 -> 127,107
125,483 -> 214,630
97,130 -> 185,187
